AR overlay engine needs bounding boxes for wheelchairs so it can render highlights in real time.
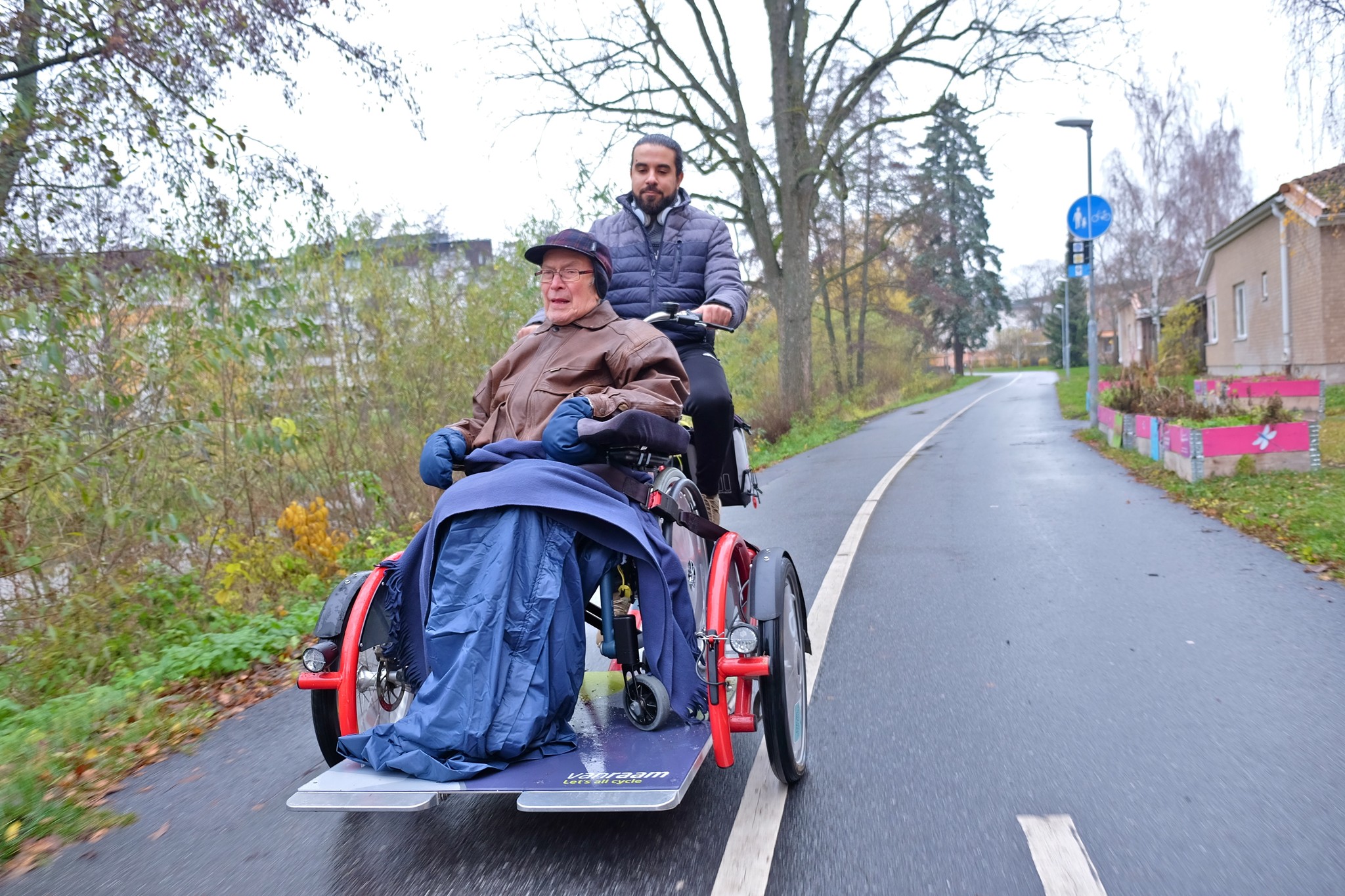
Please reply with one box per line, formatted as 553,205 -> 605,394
448,408 -> 712,733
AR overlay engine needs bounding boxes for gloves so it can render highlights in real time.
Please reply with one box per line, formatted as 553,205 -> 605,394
542,396 -> 592,464
418,427 -> 466,490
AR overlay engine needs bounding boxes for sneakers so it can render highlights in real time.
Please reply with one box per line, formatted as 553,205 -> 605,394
596,593 -> 630,646
700,494 -> 722,526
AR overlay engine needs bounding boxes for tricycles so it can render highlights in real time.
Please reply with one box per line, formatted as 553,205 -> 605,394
286,299 -> 813,814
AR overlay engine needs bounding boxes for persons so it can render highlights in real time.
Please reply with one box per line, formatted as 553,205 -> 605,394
419,229 -> 691,483
516,135 -> 748,646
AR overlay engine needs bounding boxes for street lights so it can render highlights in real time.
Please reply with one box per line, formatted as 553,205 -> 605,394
1055,119 -> 1099,429
1054,303 -> 1065,368
1055,276 -> 1070,377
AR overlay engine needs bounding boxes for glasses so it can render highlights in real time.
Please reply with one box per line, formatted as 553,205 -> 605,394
534,268 -> 595,283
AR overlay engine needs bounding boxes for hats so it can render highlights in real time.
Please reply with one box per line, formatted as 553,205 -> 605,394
524,228 -> 613,283
